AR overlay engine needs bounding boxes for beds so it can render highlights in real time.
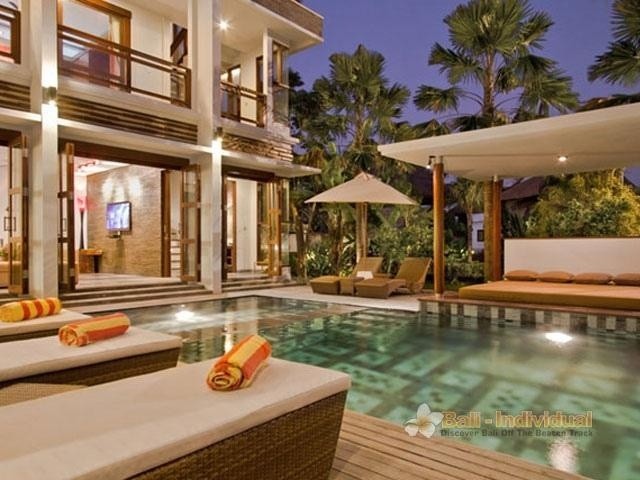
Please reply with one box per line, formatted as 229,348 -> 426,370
459,235 -> 640,308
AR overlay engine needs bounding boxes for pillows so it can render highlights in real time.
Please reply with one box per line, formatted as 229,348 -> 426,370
537,271 -> 571,283
613,273 -> 640,286
572,273 -> 611,285
503,269 -> 535,281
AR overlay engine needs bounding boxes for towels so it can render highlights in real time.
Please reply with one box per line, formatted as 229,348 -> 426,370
207,334 -> 271,391
59,312 -> 131,347
0,296 -> 61,323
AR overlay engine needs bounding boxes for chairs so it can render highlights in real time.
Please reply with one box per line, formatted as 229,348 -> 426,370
357,257 -> 432,297
310,256 -> 382,294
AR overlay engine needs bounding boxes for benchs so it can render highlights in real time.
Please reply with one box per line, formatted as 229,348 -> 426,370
0,326 -> 183,388
0,356 -> 352,480
0,307 -> 91,343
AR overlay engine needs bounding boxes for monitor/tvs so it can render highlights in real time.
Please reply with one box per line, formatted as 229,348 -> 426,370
106,202 -> 131,231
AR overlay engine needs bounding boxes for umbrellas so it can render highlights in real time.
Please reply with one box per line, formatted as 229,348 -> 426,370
305,173 -> 420,264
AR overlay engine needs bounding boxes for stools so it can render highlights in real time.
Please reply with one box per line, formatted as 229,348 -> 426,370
0,260 -> 20,288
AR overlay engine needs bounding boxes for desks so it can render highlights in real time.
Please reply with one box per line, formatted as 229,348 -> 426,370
339,273 -> 390,294
